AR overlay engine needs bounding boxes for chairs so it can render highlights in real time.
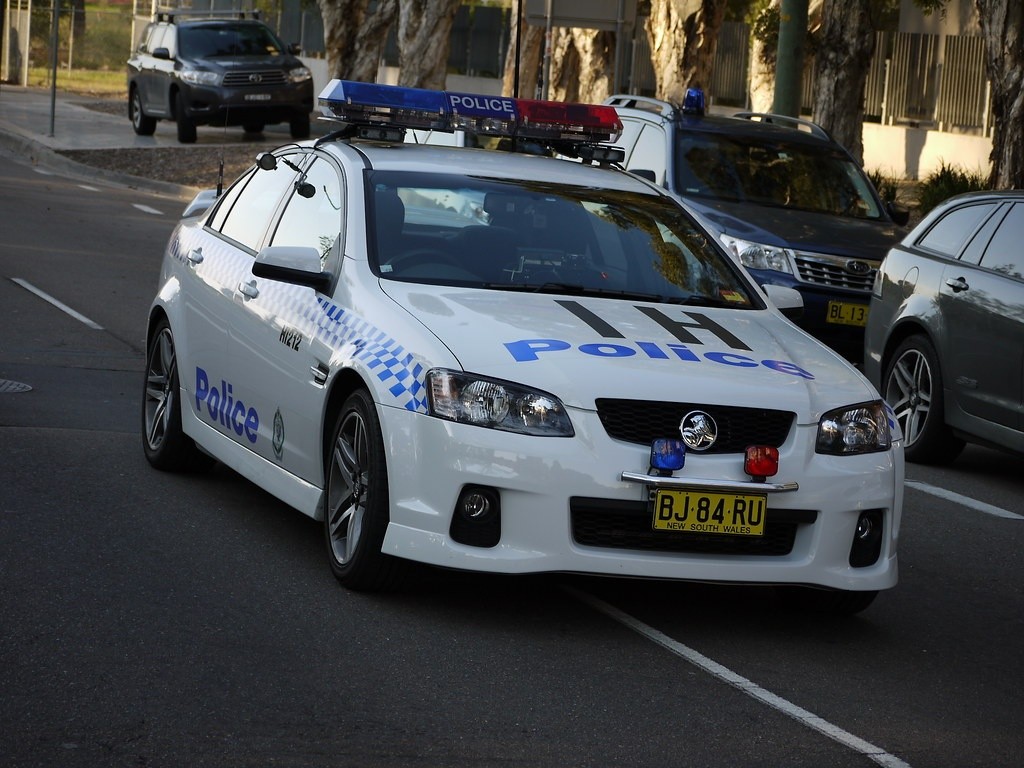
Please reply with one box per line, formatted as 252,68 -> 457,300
445,226 -> 518,268
486,190 -> 541,230
372,190 -> 405,256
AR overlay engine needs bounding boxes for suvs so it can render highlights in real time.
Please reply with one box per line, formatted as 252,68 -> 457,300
126,9 -> 314,142
551,94 -> 910,358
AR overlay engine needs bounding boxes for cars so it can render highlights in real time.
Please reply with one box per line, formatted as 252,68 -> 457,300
867,189 -> 1023,460
141,80 -> 906,605
